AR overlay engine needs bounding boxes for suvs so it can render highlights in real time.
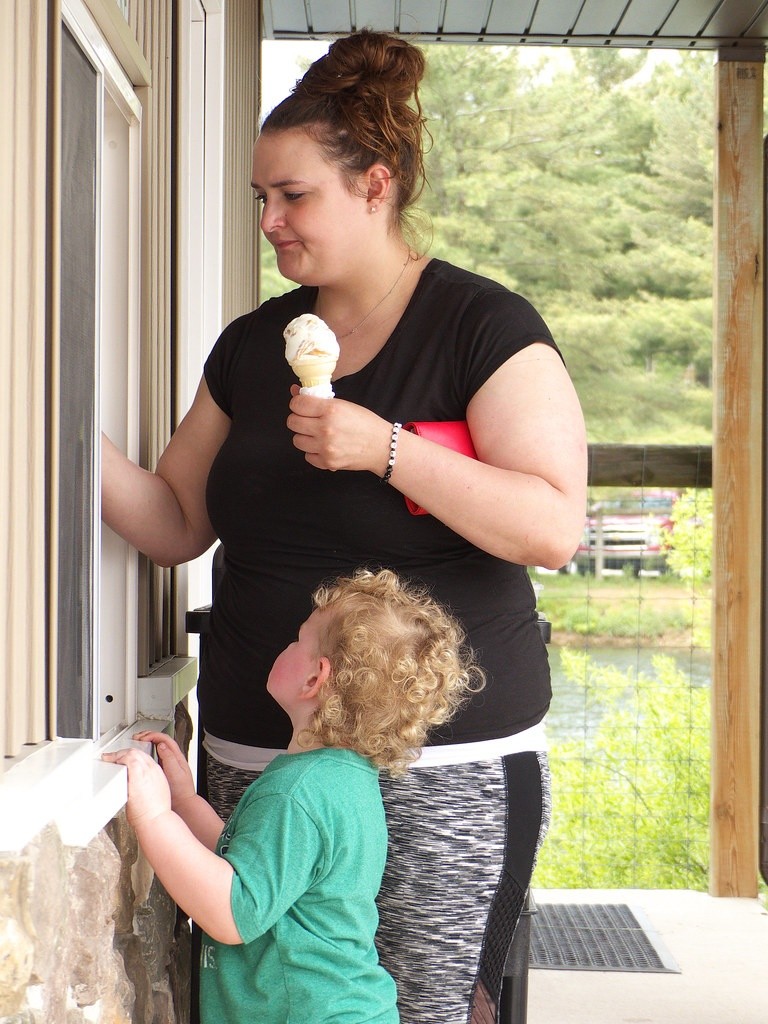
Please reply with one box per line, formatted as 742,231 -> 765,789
571,488 -> 683,575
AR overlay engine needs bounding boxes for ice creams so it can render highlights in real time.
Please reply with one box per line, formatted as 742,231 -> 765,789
283,313 -> 340,388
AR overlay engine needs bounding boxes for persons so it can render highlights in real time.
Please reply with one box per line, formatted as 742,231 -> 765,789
102,31 -> 586,1023
105,565 -> 485,1024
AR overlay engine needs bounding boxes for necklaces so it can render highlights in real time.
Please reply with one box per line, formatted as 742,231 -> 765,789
318,242 -> 411,337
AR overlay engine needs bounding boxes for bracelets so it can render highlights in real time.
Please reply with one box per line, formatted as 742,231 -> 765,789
380,422 -> 402,485
